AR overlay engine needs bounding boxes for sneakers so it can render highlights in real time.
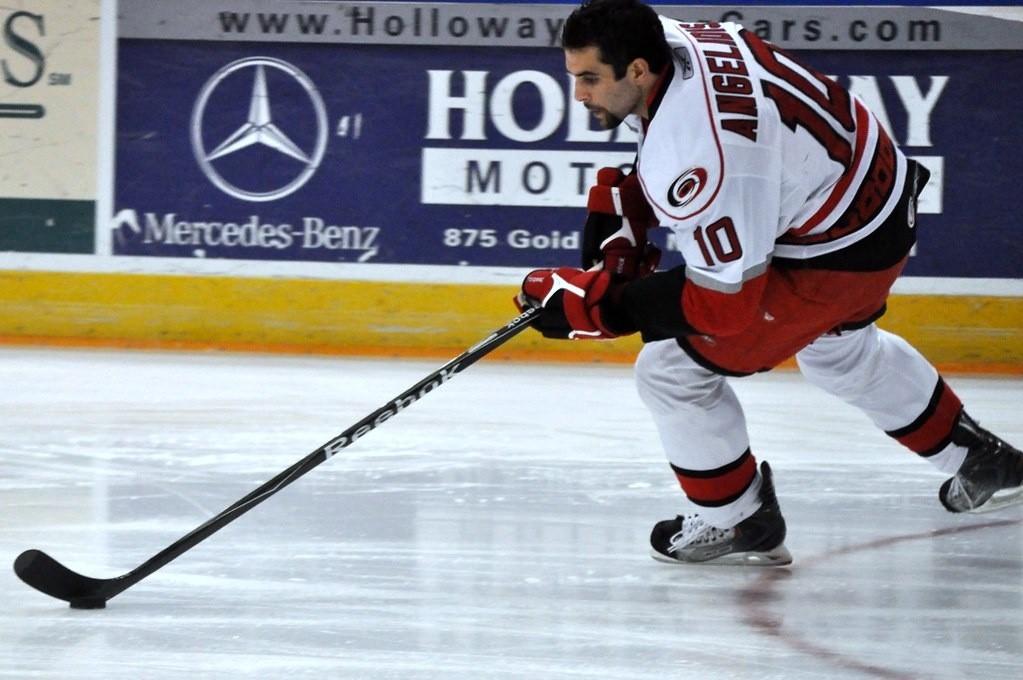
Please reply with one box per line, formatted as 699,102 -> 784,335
938,413 -> 1023,514
649,462 -> 793,566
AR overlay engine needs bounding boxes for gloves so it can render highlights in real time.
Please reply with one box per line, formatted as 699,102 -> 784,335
514,267 -> 637,341
579,165 -> 662,280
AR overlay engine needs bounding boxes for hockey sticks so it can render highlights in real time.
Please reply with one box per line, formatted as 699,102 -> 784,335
9,255 -> 605,603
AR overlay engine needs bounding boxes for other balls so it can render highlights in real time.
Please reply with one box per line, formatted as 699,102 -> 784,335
71,599 -> 109,611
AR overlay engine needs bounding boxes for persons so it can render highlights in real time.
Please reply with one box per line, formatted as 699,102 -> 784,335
514,0 -> 1023,566
